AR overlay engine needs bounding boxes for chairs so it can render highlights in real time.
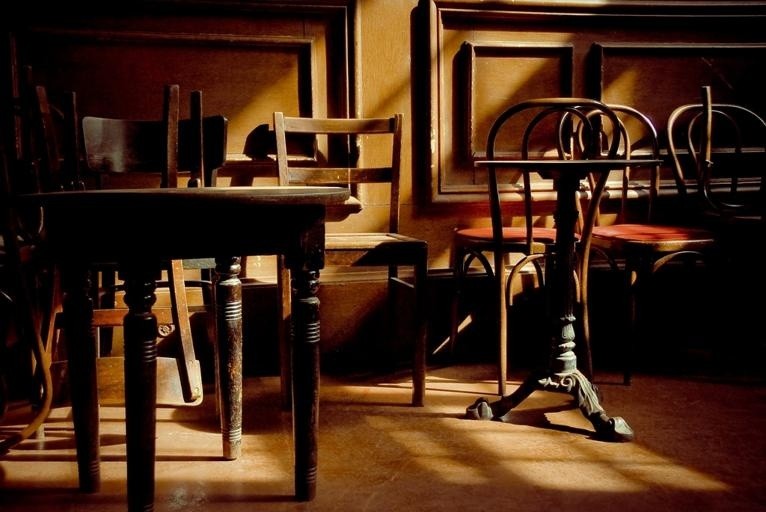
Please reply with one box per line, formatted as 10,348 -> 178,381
80,114 -> 230,428
666,104 -> 765,241
450,99 -> 621,395
273,112 -> 429,409
0,63 -> 53,456
26,85 -> 206,407
556,103 -> 714,383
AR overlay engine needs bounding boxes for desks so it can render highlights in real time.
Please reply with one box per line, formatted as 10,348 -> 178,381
465,157 -> 664,443
24,185 -> 363,512
